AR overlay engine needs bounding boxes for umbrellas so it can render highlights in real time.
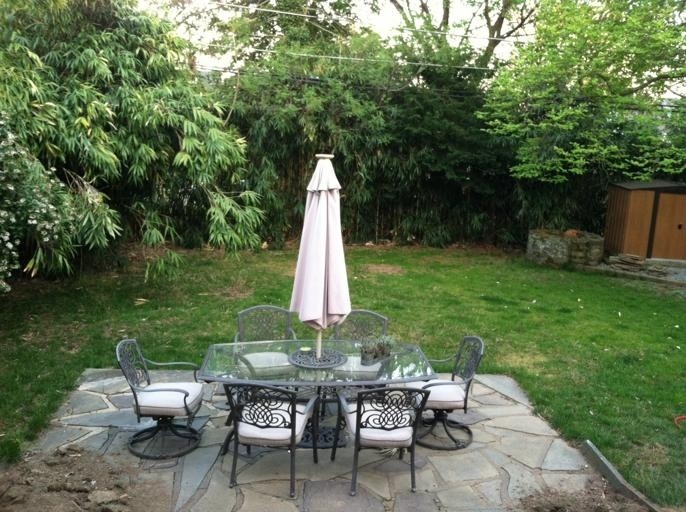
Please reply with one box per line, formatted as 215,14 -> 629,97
287,151 -> 352,358
